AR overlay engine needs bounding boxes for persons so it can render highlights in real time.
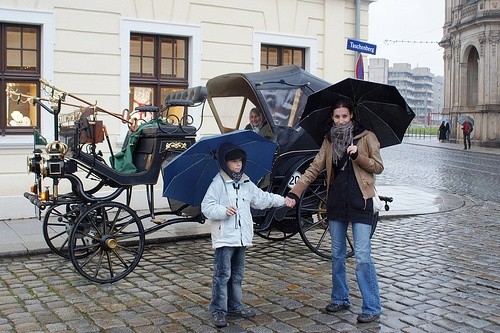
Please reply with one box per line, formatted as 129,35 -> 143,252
243,108 -> 280,191
438,120 -> 450,142
201,142 -> 297,327
461,116 -> 473,150
287,101 -> 384,322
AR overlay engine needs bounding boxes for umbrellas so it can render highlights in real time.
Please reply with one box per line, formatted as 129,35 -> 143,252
438,117 -> 451,122
297,77 -> 416,149
457,114 -> 474,125
161,129 -> 279,213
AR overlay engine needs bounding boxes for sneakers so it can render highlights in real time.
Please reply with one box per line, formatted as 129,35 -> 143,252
229,307 -> 256,318
357,312 -> 382,323
325,303 -> 350,312
211,310 -> 227,327
